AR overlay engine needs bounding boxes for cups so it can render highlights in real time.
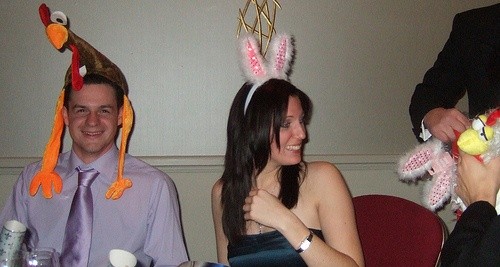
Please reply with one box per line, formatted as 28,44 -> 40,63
23,247 -> 59,267
0,220 -> 26,267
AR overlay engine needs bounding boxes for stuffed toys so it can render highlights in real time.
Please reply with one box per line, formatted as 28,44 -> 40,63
395,136 -> 460,212
452,106 -> 500,221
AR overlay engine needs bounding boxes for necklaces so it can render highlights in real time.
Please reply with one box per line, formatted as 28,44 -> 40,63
252,179 -> 280,234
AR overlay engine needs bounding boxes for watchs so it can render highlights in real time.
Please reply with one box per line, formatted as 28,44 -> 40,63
296,229 -> 314,254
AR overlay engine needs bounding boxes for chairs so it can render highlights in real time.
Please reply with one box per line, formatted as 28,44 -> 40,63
351,194 -> 447,267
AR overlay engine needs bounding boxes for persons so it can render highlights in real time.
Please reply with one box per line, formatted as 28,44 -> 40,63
211,78 -> 365,267
0,72 -> 190,267
408,3 -> 500,144
438,147 -> 500,267
